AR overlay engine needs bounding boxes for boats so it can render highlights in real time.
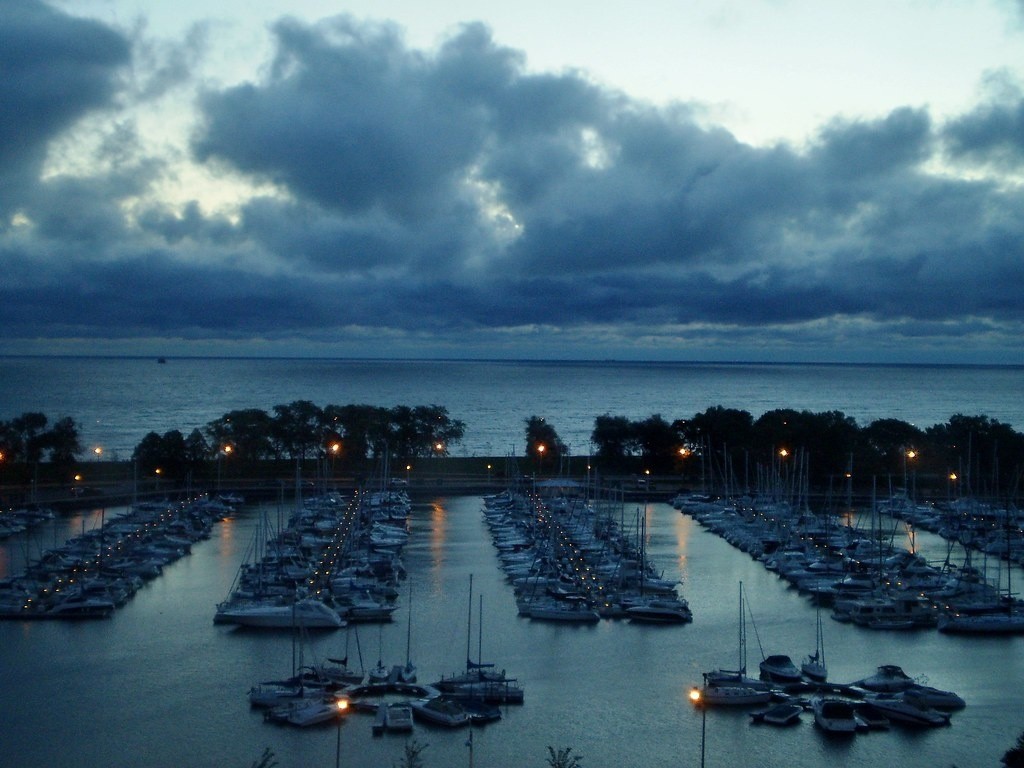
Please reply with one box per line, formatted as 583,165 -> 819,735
752,697 -> 804,724
812,696 -> 891,735
758,653 -> 802,682
375,698 -> 417,734
690,684 -> 773,704
857,663 -> 964,727
416,693 -> 473,726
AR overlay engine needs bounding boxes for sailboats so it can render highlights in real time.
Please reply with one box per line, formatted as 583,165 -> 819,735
873,428 -> 1024,566
248,570 -> 524,736
214,430 -> 412,628
667,433 -> 1024,632
805,579 -> 830,680
703,582 -> 776,690
480,442 -> 694,623
0,442 -> 244,616
